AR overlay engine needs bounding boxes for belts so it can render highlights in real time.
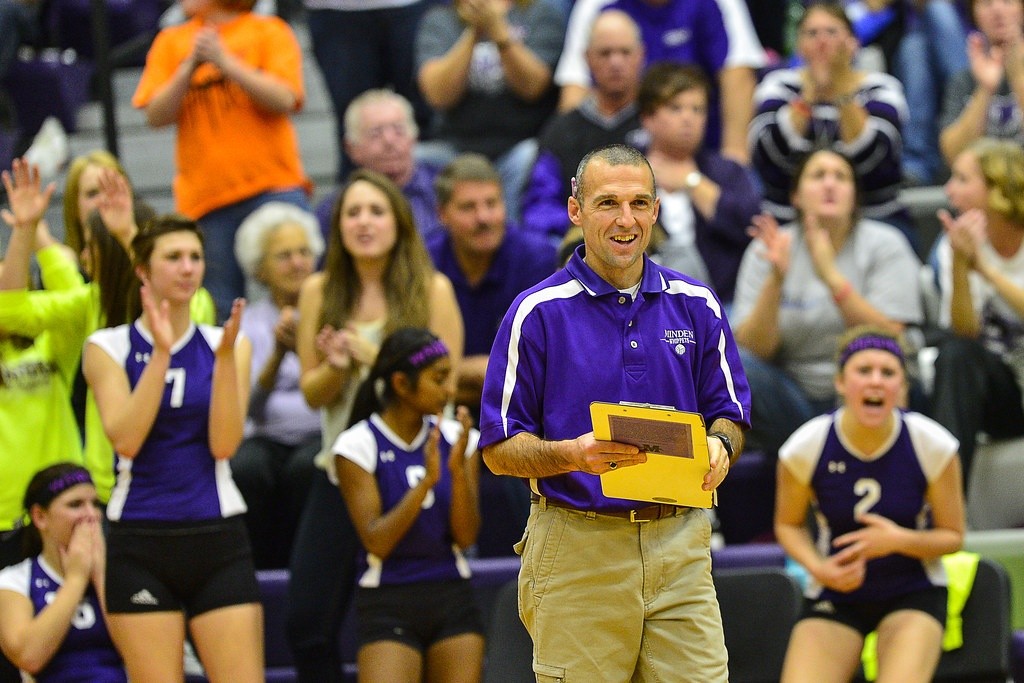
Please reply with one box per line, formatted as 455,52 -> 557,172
531,492 -> 688,524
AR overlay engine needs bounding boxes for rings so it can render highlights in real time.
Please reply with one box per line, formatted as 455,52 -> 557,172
610,462 -> 617,470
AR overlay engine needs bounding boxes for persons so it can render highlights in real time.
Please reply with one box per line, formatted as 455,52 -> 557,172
937,0 -> 1024,183
296,169 -> 464,572
729,147 -> 929,460
82,215 -> 265,683
595,62 -> 760,311
0,0 -> 1023,294
0,150 -> 215,569
0,463 -> 129,683
229,201 -> 333,551
926,135 -> 1024,447
421,152 -> 561,419
330,326 -> 484,683
747,3 -> 921,263
773,325 -> 965,683
478,144 -> 751,683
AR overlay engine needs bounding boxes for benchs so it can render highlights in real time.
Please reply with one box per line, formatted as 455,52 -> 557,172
948,433 -> 1024,533
889,182 -> 953,257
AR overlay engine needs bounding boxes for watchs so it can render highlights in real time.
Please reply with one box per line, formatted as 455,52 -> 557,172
684,168 -> 704,193
711,431 -> 733,462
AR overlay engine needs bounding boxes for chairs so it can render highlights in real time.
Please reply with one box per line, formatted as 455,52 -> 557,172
930,560 -> 1010,683
482,580 -> 536,683
711,570 -> 802,683
